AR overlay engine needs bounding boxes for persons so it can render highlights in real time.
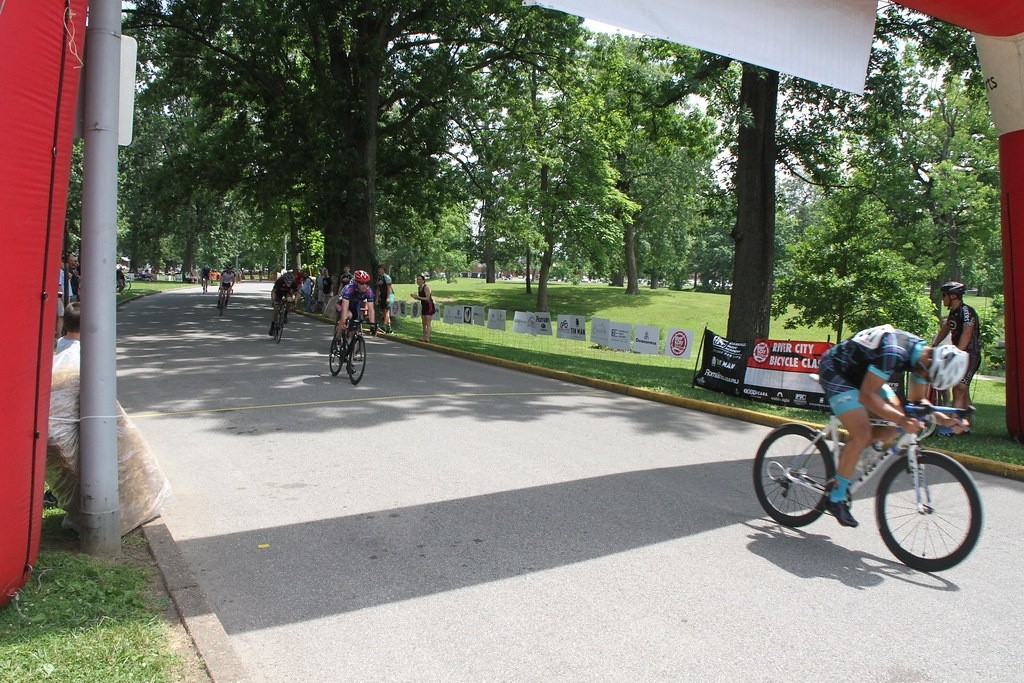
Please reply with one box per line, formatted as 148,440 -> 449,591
116,266 -> 124,293
933,281 -> 981,433
55,254 -> 81,345
200,265 -> 211,292
268,265 -> 332,336
330,270 -> 377,372
217,264 -> 235,309
52,302 -> 80,373
338,265 -> 354,295
375,264 -> 395,334
411,275 -> 435,342
819,324 -> 970,528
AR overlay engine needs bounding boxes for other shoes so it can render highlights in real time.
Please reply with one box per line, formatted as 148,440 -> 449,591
346,364 -> 356,373
824,497 -> 858,527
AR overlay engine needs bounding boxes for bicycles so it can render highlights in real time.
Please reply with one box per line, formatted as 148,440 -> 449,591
330,319 -> 378,385
218,288 -> 234,317
271,299 -> 295,344
752,400 -> 983,573
299,297 -> 307,312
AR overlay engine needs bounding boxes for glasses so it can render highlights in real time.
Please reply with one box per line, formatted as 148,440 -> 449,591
357,281 -> 368,285
942,292 -> 951,297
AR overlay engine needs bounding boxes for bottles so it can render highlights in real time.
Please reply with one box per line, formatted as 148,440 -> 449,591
344,328 -> 348,342
345,338 -> 352,348
854,441 -> 885,474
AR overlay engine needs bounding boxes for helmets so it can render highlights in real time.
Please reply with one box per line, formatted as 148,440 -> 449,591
940,281 -> 967,300
354,270 -> 371,282
928,345 -> 969,390
